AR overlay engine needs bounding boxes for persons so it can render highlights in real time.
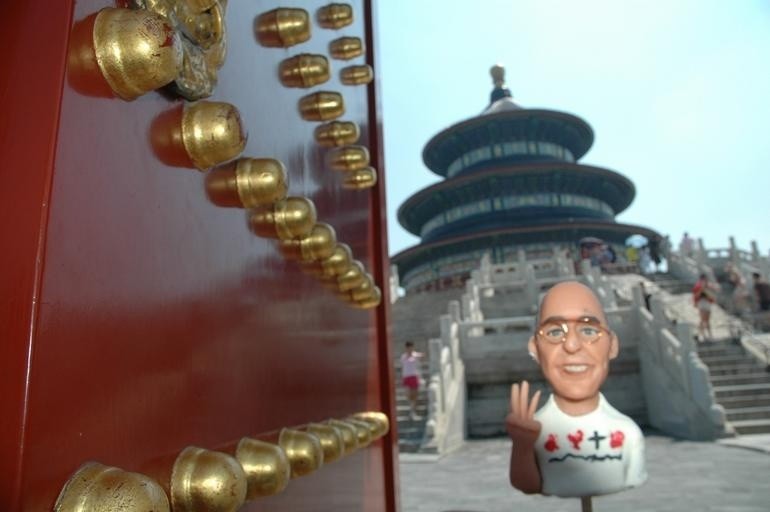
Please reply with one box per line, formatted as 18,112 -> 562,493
678,231 -> 696,258
691,260 -> 770,345
540,229 -> 672,275
399,341 -> 427,421
504,280 -> 650,499
